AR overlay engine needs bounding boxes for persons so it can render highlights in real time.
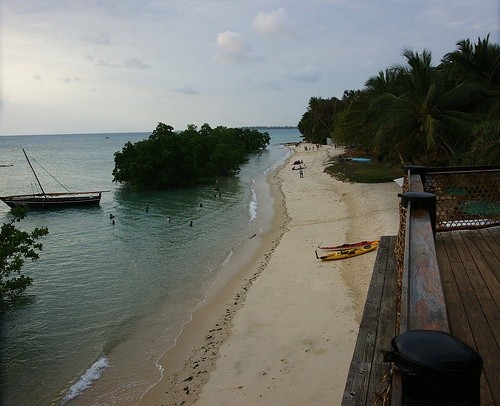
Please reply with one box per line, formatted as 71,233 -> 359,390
112,220 -> 115,224
168,217 -> 171,221
304,142 -> 320,151
146,206 -> 148,212
200,203 -> 203,207
300,170 -> 303,178
109,214 -> 115,219
214,184 -> 222,197
190,221 -> 193,227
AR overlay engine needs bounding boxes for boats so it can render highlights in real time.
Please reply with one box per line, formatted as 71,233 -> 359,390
258,147 -> 263,156
0,148 -> 102,211
315,240 -> 380,262
